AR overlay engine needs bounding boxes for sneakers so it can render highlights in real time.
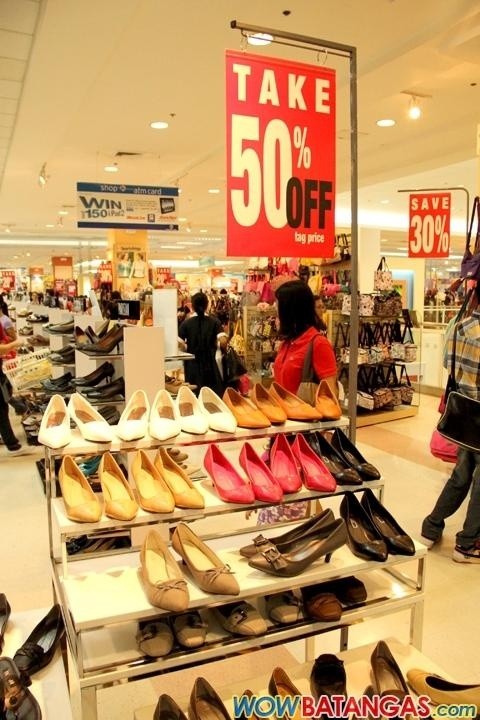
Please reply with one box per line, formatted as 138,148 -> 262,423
419,528 -> 480,565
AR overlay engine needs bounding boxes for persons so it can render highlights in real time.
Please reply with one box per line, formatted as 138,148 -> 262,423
103,291 -> 125,320
0,295 -> 16,442
117,253 -> 131,277
420,280 -> 480,563
273,280 -> 338,400
178,292 -> 229,397
129,253 -> 146,278
0,322 -> 36,455
313,295 -> 323,320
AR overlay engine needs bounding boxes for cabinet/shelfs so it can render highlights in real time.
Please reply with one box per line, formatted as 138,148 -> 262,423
4,287 -> 199,411
242,302 -> 422,427
39,422 -> 454,718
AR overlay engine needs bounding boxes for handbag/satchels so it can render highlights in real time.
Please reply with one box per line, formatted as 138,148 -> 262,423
430,429 -> 462,464
221,346 -> 247,380
251,233 -> 419,413
438,389 -> 479,452
460,248 -> 480,282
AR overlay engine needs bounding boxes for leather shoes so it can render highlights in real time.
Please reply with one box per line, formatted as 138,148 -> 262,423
270,381 -> 323,424
406,667 -> 480,720
0,592 -> 65,720
116,389 -> 150,442
176,385 -> 210,435
197,386 -> 237,434
150,386 -> 177,441
268,666 -> 308,719
369,640 -> 411,720
316,379 -> 342,421
222,386 -> 271,430
310,653 -> 351,719
144,692 -> 187,719
247,382 -> 286,424
37,392 -> 71,451
236,689 -> 264,720
51,428 -> 416,657
65,391 -> 114,443
188,677 -> 232,720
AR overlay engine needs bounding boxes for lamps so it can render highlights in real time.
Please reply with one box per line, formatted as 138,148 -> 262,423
408,95 -> 422,119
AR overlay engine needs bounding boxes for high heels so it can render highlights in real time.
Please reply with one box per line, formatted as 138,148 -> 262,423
0,310 -> 125,452
